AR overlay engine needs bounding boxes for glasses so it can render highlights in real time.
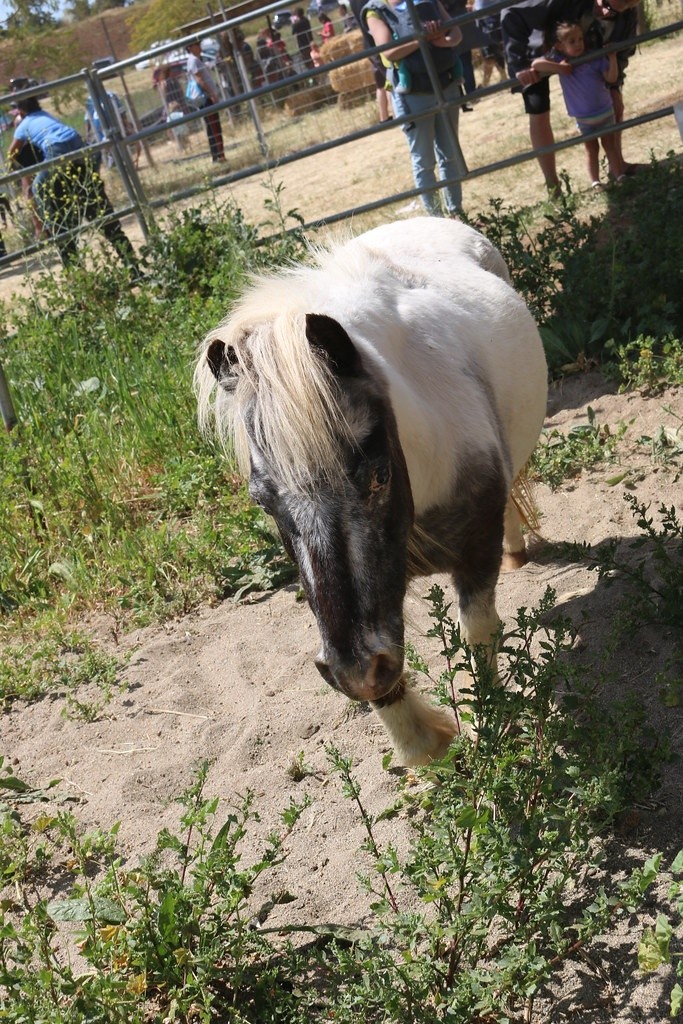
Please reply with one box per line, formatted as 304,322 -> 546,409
602,0 -> 619,15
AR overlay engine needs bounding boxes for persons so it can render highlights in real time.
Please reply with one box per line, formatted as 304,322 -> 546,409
9,98 -> 87,222
184,38 -> 228,164
360,1 -> 472,227
530,21 -> 627,188
500,0 -> 650,202
156,0 -> 513,155
7,110 -> 144,283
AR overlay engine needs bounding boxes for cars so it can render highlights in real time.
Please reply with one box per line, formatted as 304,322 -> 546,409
135,34 -> 224,91
9,77 -> 47,101
304,0 -> 340,21
270,10 -> 293,31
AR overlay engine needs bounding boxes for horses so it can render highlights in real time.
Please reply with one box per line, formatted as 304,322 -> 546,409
188,216 -> 548,767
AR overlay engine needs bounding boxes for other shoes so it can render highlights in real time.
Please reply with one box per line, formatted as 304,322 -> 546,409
625,165 -> 638,176
592,181 -> 602,190
452,213 -> 469,224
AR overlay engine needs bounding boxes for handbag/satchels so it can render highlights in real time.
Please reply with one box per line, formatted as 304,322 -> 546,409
185,82 -> 206,107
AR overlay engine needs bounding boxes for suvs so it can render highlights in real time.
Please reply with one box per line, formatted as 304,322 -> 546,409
92,57 -> 122,78
